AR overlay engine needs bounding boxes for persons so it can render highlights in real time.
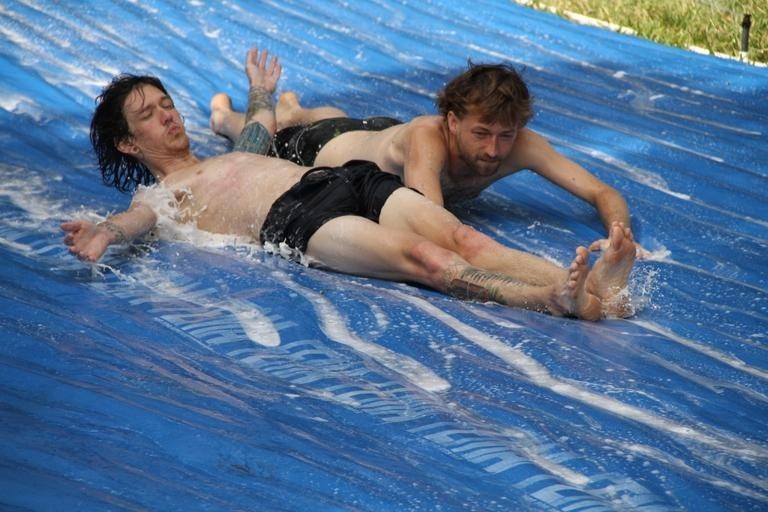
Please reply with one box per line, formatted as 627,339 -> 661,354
59,46 -> 637,321
210,57 -> 652,259
